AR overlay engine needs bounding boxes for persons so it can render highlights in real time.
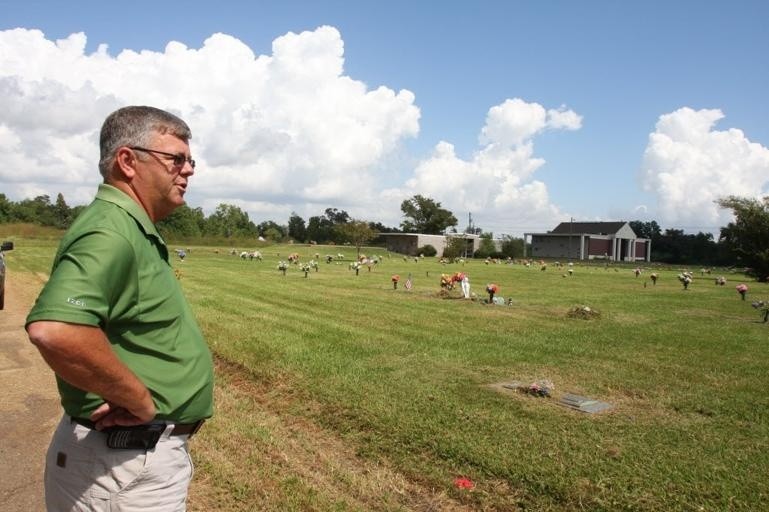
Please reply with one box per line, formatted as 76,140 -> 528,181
24,105 -> 215,512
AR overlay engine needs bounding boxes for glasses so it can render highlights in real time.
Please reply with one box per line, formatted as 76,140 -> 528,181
130,147 -> 196,169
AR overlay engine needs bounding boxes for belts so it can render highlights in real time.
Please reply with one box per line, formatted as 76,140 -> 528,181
75,417 -> 205,437
